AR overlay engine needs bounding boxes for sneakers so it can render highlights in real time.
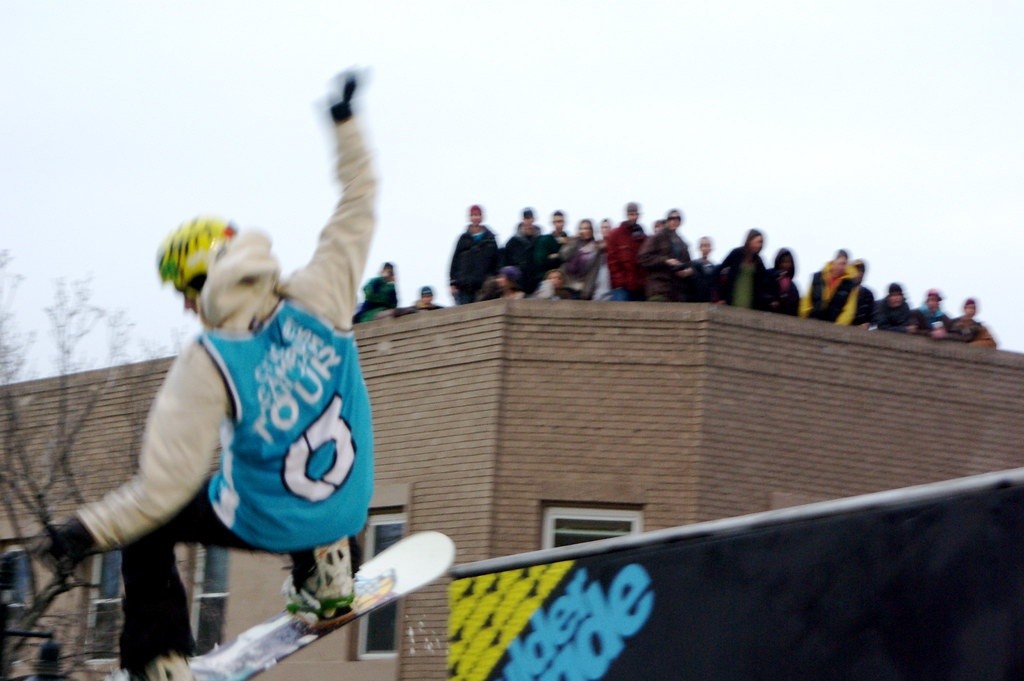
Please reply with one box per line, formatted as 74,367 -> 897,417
282,538 -> 361,625
136,652 -> 196,681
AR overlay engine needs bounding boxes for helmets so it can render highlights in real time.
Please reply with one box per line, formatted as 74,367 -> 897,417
153,217 -> 237,312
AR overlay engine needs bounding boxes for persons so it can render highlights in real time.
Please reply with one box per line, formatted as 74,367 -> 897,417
910,288 -> 950,338
951,298 -> 996,348
765,247 -> 800,317
481,265 -> 528,301
874,282 -> 918,333
28,67 -> 381,681
504,201 -> 691,303
717,229 -> 767,304
351,261 -> 397,325
692,237 -> 716,303
800,250 -> 875,329
449,205 -> 498,304
411,287 -> 443,312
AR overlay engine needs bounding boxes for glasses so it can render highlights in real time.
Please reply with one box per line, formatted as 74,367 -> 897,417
667,215 -> 681,220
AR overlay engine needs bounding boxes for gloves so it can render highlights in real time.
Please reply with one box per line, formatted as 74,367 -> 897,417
330,76 -> 358,123
36,516 -> 97,571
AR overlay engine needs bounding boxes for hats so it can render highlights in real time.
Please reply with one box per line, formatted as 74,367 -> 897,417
421,286 -> 432,295
501,265 -> 522,284
927,289 -> 942,301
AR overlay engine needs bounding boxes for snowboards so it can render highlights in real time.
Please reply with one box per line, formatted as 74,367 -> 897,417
117,531 -> 457,680
